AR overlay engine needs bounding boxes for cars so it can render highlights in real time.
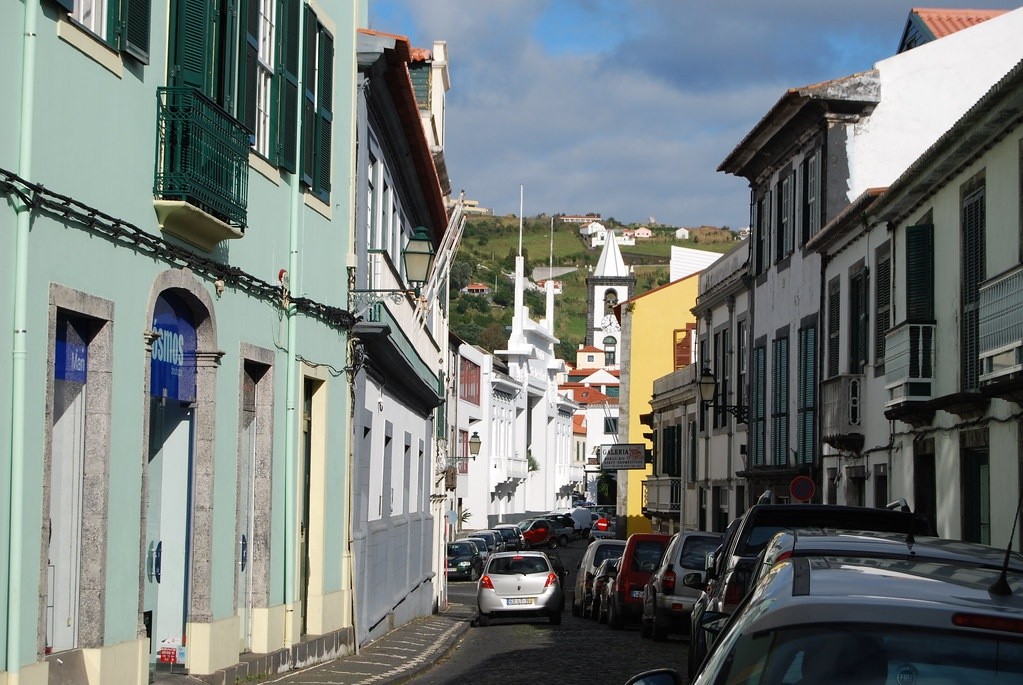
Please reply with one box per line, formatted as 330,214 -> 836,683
456,536 -> 490,571
599,557 -> 620,626
516,518 -> 559,549
607,516 -> 617,527
526,549 -> 569,612
570,491 -> 594,508
642,531 -> 726,641
496,524 -> 526,549
448,540 -> 483,582
749,529 -> 1022,602
492,527 -> 522,548
468,532 -> 500,556
588,520 -> 617,543
481,529 -> 507,554
624,558 -> 1019,685
476,550 -> 563,623
583,558 -> 621,620
591,511 -> 610,525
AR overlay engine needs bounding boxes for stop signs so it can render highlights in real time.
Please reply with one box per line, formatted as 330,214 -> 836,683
596,518 -> 609,531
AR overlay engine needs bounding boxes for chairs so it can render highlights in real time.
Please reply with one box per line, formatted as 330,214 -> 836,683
800,632 -> 889,685
511,561 -> 530,572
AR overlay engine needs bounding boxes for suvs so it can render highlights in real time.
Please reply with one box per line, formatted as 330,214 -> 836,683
534,514 -> 576,548
686,489 -> 939,685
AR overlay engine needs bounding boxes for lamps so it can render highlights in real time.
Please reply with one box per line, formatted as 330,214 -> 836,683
696,367 -> 717,406
402,228 -> 436,297
470,432 -> 480,454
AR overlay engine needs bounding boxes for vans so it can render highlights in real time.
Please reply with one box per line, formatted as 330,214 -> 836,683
573,537 -> 627,617
550,507 -> 592,541
610,533 -> 674,629
